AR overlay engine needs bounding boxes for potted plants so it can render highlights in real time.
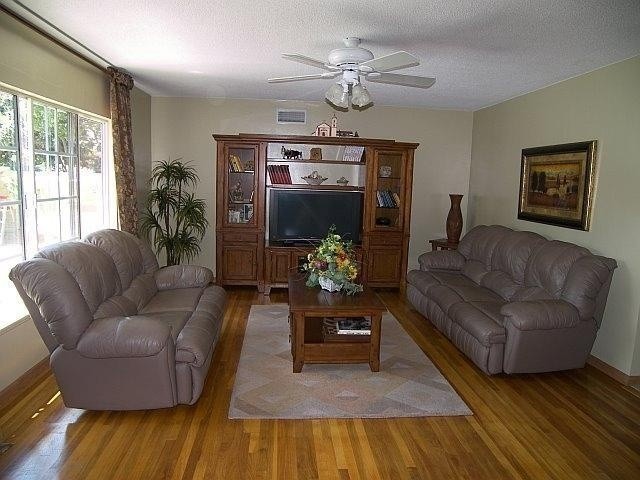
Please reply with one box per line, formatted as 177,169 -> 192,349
136,158 -> 210,266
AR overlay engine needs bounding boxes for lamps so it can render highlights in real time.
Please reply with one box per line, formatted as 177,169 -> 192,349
336,70 -> 360,97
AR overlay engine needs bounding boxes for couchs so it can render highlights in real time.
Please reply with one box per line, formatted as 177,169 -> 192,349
8,228 -> 229,412
402,225 -> 619,384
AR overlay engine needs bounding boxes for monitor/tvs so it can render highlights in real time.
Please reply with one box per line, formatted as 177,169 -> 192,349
265,187 -> 365,245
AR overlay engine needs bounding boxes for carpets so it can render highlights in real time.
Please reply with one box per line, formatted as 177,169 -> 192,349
225,302 -> 474,421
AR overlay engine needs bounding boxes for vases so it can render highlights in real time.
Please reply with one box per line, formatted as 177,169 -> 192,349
319,274 -> 350,295
444,192 -> 467,246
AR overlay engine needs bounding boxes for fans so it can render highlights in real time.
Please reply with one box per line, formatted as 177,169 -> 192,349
265,37 -> 439,114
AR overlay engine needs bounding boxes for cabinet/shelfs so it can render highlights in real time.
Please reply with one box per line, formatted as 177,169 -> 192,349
210,131 -> 421,299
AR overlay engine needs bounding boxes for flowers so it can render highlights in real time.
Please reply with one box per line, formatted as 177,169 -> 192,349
301,223 -> 365,297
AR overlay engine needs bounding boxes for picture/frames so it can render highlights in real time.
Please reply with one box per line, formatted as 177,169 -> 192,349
512,139 -> 601,232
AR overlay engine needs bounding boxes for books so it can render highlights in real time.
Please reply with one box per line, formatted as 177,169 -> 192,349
268,165 -> 292,184
336,319 -> 372,335
343,145 -> 365,162
